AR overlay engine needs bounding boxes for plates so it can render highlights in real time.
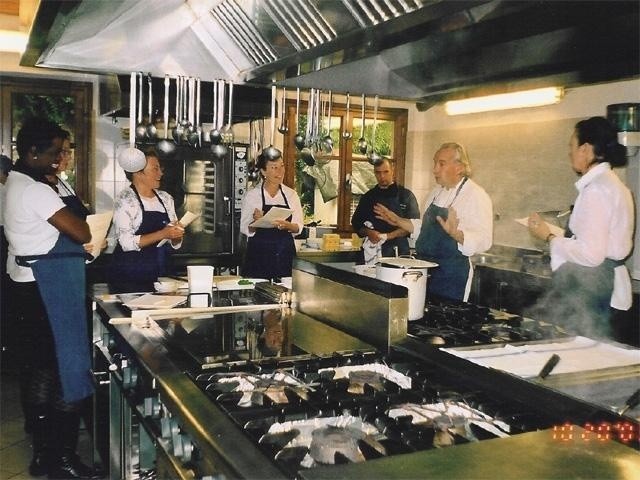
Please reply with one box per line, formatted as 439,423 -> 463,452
218,279 -> 269,290
124,293 -> 187,311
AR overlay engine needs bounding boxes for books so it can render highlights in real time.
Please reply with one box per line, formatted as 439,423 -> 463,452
84,211 -> 114,266
248,206 -> 295,231
517,211 -> 567,244
154,209 -> 203,249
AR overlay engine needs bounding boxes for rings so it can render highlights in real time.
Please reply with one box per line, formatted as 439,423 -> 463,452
280,224 -> 282,227
277,226 -> 281,229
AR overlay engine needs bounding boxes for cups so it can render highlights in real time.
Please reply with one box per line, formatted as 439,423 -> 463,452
187,266 -> 215,300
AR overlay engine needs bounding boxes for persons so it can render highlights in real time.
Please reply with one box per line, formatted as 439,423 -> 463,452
1,114 -> 101,480
373,141 -> 494,305
527,113 -> 636,344
237,143 -> 308,278
19,128 -> 109,434
106,151 -> 189,294
0,154 -> 11,230
350,153 -> 422,264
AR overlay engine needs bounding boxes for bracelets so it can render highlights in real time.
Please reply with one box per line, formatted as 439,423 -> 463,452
545,233 -> 555,242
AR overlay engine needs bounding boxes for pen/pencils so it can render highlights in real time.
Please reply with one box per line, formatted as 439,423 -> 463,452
154,300 -> 166,305
556,210 -> 571,218
163,221 -> 185,231
253,211 -> 264,215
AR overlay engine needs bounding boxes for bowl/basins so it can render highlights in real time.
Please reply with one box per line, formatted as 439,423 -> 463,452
155,282 -> 179,292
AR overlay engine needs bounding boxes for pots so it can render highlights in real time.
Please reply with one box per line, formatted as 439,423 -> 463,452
377,261 -> 428,322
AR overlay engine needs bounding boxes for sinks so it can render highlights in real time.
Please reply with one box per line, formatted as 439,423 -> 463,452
470,255 -> 503,264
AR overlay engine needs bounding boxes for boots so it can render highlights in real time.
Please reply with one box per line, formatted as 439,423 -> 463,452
47,421 -> 93,479
21,414 -> 52,478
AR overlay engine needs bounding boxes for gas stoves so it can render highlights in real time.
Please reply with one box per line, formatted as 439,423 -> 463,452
404,298 -> 571,347
187,351 -> 549,473
